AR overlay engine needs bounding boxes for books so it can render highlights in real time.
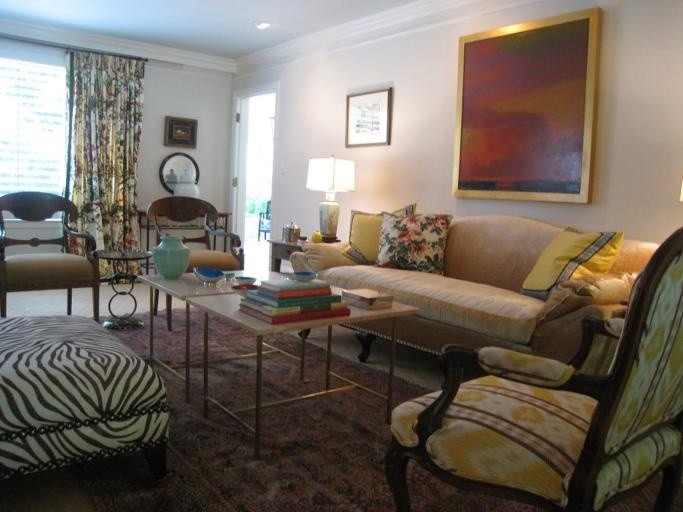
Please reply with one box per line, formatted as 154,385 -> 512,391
258,287 -> 332,298
239,297 -> 348,316
262,278 -> 331,291
238,303 -> 351,322
341,287 -> 394,309
247,290 -> 341,306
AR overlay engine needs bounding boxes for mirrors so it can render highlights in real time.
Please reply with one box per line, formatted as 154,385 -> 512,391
160,152 -> 199,194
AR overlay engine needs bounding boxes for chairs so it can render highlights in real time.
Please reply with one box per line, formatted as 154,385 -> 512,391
385,227 -> 683,512
147,195 -> 243,330
257,200 -> 272,243
1,191 -> 100,323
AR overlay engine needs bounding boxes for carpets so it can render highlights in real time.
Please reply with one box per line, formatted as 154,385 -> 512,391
89,303 -> 540,511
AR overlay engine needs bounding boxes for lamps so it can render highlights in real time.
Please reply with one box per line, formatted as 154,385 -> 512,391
305,157 -> 356,244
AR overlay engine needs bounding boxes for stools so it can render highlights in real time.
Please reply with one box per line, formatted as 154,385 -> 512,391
0,315 -> 167,502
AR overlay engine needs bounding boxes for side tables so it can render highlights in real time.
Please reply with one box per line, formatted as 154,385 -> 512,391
91,249 -> 152,330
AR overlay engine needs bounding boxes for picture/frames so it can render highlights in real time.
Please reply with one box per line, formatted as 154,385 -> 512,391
345,87 -> 391,147
452,7 -> 603,204
164,116 -> 198,148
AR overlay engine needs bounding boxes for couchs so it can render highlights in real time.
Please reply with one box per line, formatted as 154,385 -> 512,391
291,216 -> 658,377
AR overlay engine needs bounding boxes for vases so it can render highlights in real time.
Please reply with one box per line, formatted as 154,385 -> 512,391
153,235 -> 188,279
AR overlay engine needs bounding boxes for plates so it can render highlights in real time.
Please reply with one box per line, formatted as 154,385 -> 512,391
231,285 -> 258,295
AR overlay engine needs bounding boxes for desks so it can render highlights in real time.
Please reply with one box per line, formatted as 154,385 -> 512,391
137,272 -> 309,402
185,286 -> 417,456
139,212 -> 232,276
267,240 -> 303,274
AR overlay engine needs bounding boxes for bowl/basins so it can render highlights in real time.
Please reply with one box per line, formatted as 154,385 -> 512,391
235,276 -> 256,285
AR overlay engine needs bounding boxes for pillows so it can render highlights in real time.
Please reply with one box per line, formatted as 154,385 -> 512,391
343,203 -> 419,264
375,212 -> 453,276
520,225 -> 623,300
